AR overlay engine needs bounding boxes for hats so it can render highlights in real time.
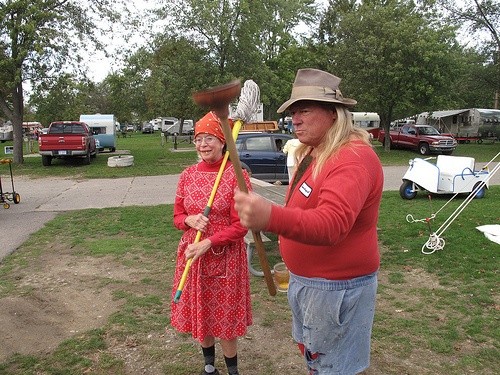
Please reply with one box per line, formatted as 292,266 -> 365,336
277,68 -> 357,114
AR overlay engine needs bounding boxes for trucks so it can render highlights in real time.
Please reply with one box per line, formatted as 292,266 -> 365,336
166,119 -> 194,135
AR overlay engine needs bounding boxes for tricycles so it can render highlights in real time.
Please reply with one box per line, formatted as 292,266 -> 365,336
399,155 -> 490,200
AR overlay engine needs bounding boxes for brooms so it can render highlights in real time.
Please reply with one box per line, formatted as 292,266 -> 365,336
193,78 -> 278,298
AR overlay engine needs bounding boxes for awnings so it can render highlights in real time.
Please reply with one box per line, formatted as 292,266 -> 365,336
426,108 -> 471,133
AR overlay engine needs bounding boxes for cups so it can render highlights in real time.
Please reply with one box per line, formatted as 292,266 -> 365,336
274,263 -> 290,293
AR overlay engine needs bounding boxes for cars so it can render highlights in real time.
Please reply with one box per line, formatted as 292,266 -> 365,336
143,123 -> 154,134
127,125 -> 137,132
227,134 -> 298,185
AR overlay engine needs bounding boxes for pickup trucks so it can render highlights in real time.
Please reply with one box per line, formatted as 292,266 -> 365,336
378,124 -> 457,155
38,121 -> 98,166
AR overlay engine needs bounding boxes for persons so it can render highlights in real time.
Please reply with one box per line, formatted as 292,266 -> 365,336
116,119 -> 140,139
233,68 -> 384,375
275,139 -> 284,152
26,126 -> 49,140
169,111 -> 253,375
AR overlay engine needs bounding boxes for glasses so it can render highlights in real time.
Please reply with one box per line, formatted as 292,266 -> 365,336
192,135 -> 215,144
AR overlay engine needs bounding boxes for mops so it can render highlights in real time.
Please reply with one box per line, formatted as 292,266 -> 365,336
172,80 -> 263,304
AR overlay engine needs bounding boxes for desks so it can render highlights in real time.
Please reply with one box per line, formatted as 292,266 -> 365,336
247,185 -> 289,276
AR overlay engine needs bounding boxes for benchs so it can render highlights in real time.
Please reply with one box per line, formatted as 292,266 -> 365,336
244,230 -> 271,247
436,155 -> 476,190
